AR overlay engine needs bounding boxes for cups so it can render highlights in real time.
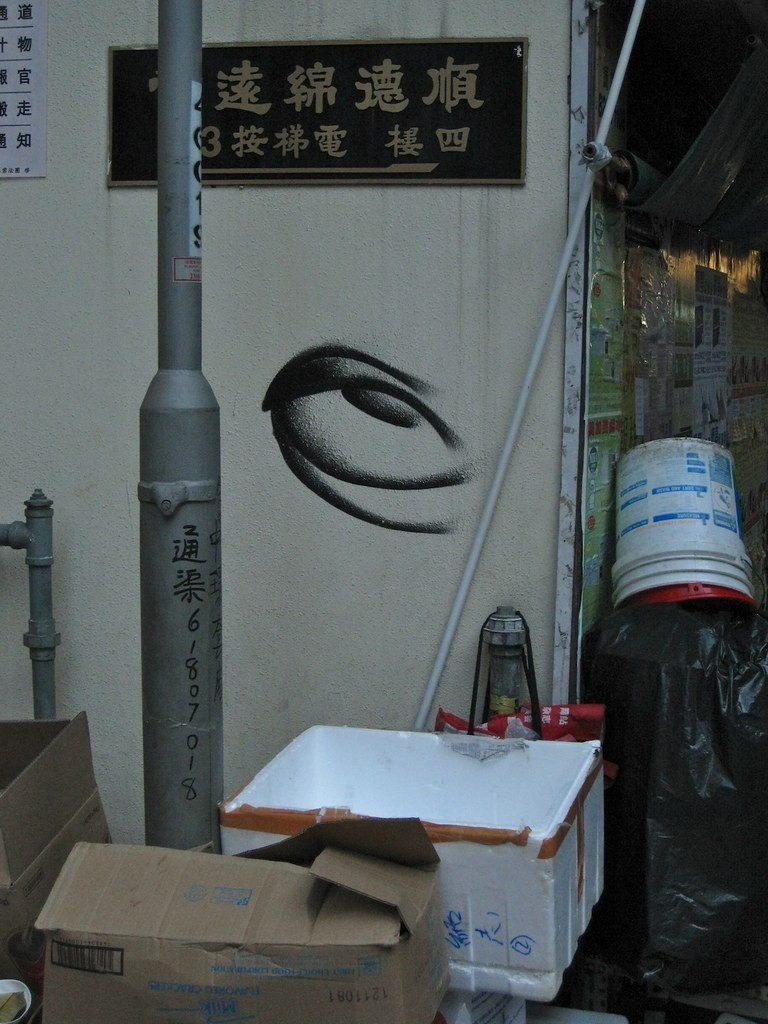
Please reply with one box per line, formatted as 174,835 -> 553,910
0,978 -> 32,1024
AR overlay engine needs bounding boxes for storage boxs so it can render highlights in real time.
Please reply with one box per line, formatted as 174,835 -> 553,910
0,707 -> 607,1024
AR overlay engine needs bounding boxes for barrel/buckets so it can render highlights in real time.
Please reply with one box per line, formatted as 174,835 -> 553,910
612,437 -> 759,616
